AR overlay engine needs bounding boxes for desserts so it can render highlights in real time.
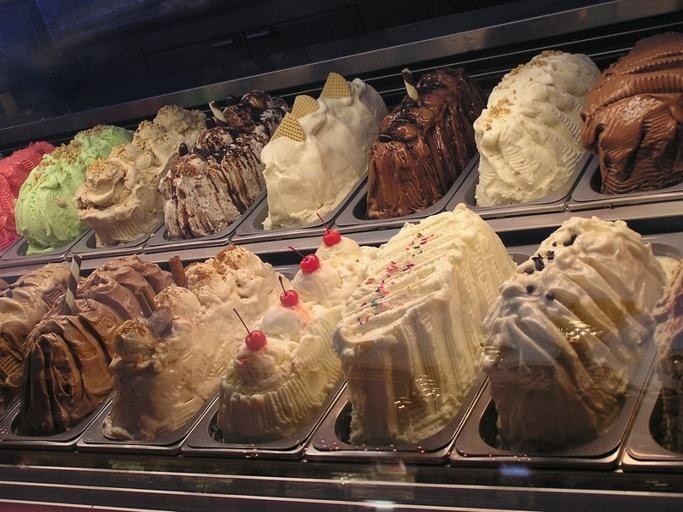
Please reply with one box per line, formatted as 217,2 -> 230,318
1,32 -> 681,254
0,203 -> 683,450
